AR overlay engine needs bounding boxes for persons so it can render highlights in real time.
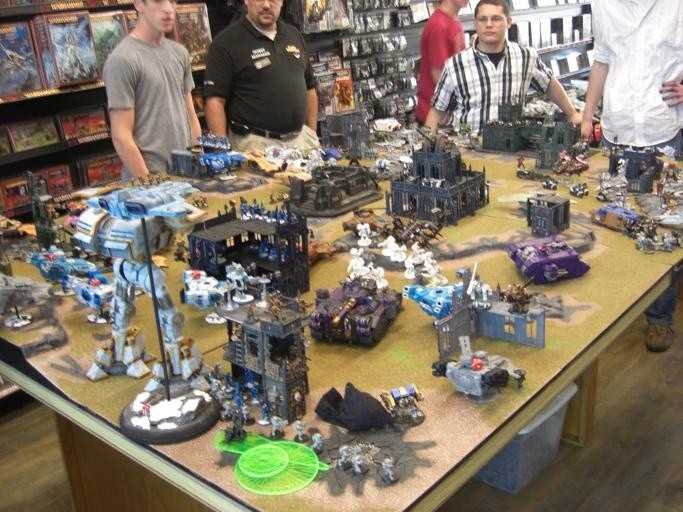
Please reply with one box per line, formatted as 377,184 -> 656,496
580,0 -> 683,355
415,1 -> 466,126
105,1 -> 202,183
424,1 -> 580,143
203,1 -> 323,156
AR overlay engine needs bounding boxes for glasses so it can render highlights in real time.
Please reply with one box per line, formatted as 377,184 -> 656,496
476,16 -> 502,26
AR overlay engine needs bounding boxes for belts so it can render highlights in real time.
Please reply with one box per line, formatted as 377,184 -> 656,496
248,127 -> 300,142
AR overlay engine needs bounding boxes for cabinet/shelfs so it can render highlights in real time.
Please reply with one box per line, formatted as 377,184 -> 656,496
1,0 -> 211,222
297,0 -> 602,130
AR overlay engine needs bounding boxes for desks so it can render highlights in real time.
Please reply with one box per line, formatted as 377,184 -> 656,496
0,135 -> 683,512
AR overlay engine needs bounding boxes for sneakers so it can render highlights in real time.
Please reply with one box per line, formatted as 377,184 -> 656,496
645,324 -> 674,351
